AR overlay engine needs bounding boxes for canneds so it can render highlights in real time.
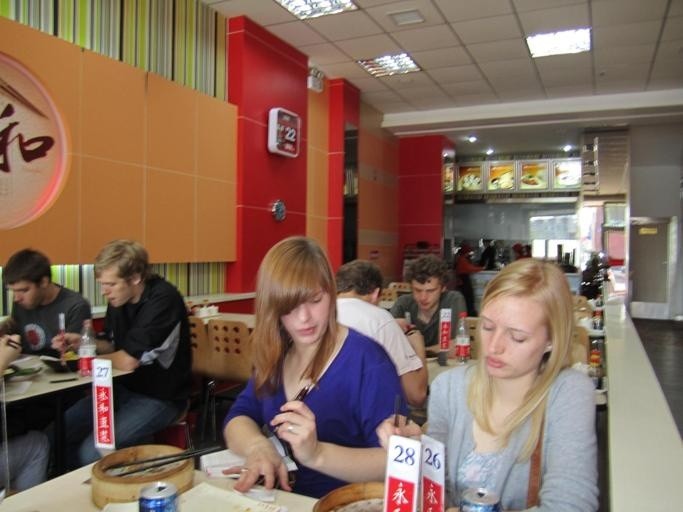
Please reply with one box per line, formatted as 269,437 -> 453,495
137,482 -> 178,511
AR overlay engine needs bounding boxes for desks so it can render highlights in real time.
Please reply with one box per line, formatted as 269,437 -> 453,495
201,311 -> 258,331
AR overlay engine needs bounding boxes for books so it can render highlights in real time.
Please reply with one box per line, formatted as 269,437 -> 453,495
195,433 -> 304,482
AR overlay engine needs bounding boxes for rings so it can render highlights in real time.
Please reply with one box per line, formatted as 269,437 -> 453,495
240,467 -> 249,473
288,424 -> 294,433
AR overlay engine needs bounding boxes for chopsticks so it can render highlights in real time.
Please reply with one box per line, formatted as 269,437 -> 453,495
0,334 -> 24,349
405,415 -> 410,427
106,446 -> 220,477
404,327 -> 416,335
59,313 -> 65,358
396,395 -> 400,426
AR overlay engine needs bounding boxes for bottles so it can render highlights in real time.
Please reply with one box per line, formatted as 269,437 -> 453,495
588,351 -> 603,390
594,300 -> 603,330
455,312 -> 470,363
80,318 -> 96,377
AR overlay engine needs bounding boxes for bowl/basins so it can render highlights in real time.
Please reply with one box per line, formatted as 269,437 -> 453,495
43,350 -> 82,372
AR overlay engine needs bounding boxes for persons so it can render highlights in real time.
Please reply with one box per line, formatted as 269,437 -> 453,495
222,236 -> 412,493
0,330 -> 53,493
453,247 -> 486,317
0,247 -> 94,373
425,257 -> 600,512
386,253 -> 467,358
331,259 -> 427,406
478,239 -> 497,270
512,243 -> 531,260
63,238 -> 192,470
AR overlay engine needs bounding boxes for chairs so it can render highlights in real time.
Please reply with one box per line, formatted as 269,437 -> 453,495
204,319 -> 251,441
388,283 -> 412,294
380,289 -> 397,311
161,316 -> 207,445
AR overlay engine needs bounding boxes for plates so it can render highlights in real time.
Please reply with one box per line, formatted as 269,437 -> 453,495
7,367 -> 44,381
426,358 -> 439,362
